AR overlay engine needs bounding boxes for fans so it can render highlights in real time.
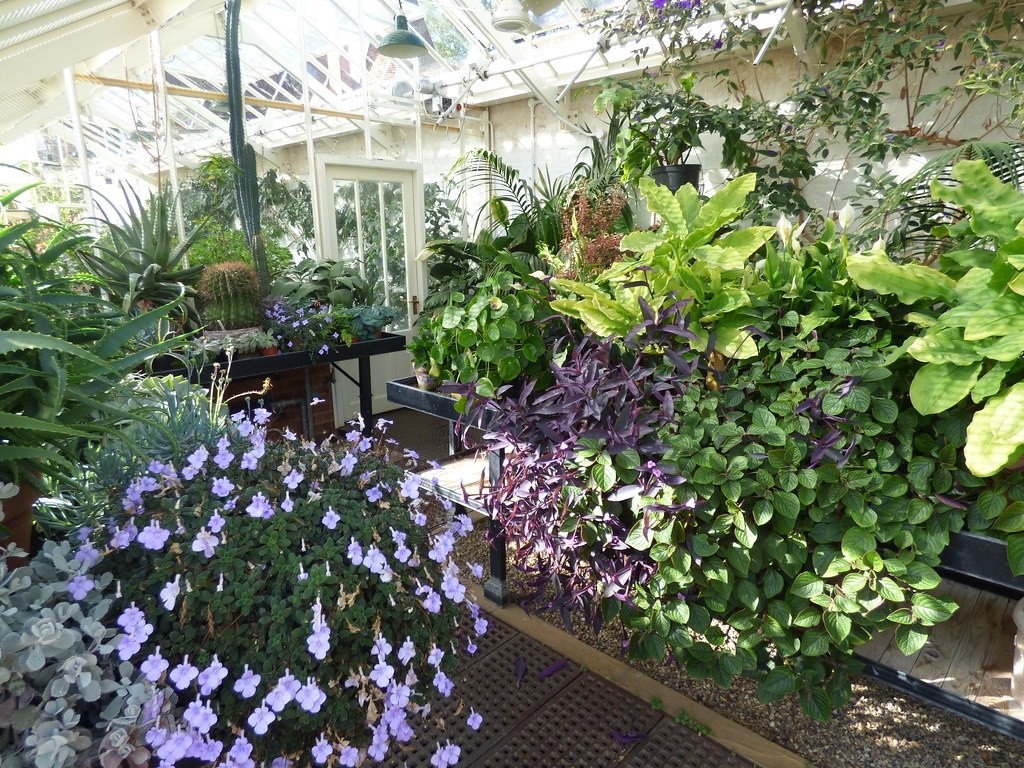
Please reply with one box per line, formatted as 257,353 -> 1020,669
463,0 -> 564,35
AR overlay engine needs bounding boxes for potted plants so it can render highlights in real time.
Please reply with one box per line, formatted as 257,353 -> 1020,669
0,162 -> 395,573
405,1 -> 1024,723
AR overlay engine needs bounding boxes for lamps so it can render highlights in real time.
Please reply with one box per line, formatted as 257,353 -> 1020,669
377,0 -> 428,60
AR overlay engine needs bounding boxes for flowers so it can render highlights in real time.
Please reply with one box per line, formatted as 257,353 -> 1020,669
68,394 -> 488,768
265,299 -> 354,362
576,1 -> 779,67
